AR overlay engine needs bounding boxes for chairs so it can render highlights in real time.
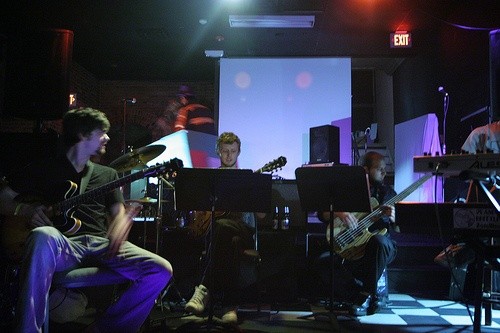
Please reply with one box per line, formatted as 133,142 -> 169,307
48,260 -> 134,333
199,215 -> 262,312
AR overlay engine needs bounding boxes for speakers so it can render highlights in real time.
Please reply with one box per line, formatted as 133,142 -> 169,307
309,125 -> 340,164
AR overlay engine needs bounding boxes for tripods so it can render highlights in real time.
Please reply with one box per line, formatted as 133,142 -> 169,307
172,167 -> 272,333
295,165 -> 373,333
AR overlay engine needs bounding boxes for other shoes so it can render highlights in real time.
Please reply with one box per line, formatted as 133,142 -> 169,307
434,244 -> 463,269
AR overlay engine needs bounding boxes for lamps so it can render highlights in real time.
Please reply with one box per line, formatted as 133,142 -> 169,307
204,50 -> 225,57
228,15 -> 315,28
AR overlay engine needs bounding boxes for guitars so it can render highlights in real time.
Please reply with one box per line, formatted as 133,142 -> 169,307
325,170 -> 434,261
185,156 -> 285,242
3,156 -> 184,260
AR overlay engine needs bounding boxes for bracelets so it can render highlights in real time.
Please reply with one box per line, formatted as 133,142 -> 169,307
14,202 -> 24,216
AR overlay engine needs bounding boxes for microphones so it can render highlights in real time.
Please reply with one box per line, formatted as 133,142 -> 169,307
438,86 -> 448,96
123,96 -> 137,103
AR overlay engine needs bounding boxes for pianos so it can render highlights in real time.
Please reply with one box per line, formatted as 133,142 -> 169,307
394,150 -> 499,333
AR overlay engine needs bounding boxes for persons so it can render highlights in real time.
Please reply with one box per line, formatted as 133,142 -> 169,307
174,81 -> 217,134
0,108 -> 174,333
316,151 -> 401,318
433,109 -> 500,268
184,132 -> 267,326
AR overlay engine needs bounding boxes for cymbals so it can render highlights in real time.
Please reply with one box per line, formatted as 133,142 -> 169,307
109,144 -> 167,170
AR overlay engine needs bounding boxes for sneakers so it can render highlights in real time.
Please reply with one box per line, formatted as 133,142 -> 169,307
185,284 -> 209,314
221,306 -> 238,326
